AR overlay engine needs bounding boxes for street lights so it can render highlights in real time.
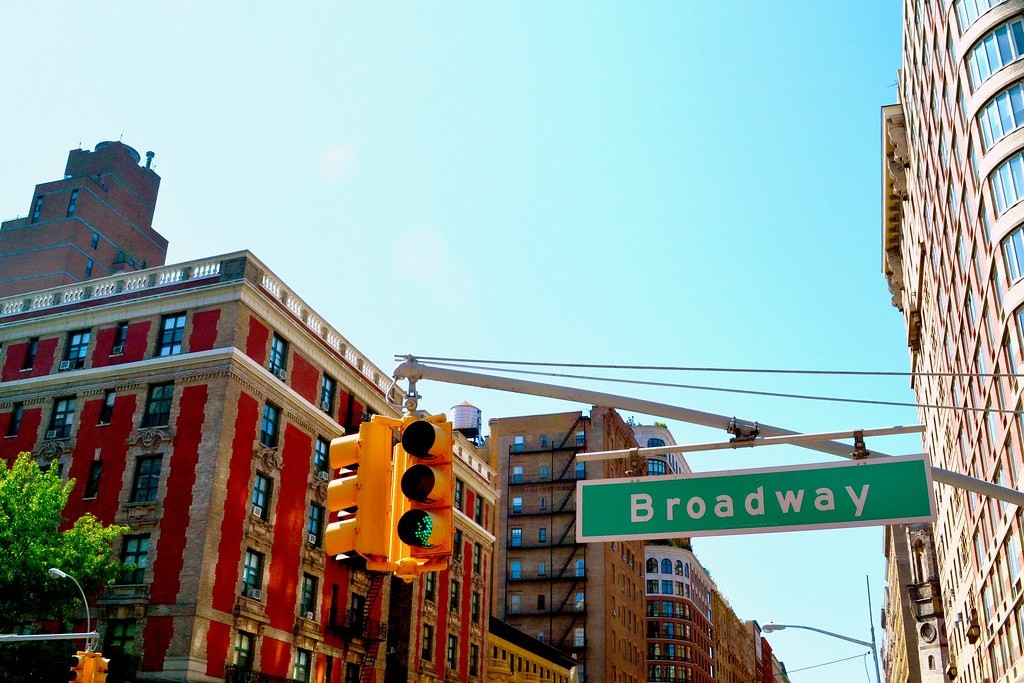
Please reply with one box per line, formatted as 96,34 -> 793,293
762,575 -> 883,683
44,567 -> 92,645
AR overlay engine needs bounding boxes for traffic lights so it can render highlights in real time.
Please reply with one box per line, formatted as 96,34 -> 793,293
396,420 -> 456,558
67,653 -> 89,683
93,653 -> 110,683
324,422 -> 393,560
